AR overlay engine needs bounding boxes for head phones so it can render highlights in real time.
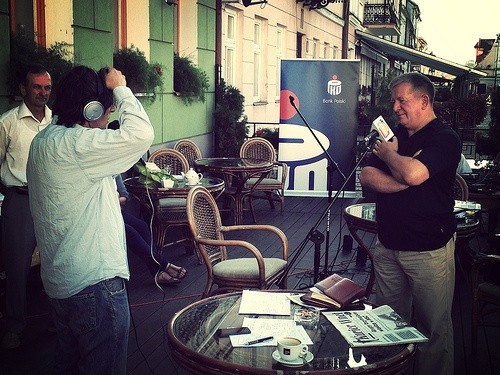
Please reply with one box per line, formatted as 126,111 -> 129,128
83,70 -> 104,122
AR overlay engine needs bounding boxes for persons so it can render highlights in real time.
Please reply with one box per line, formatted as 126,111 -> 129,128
0,69 -> 53,349
26,66 -> 154,375
116,174 -> 187,285
109,119 -> 121,129
359,73 -> 462,375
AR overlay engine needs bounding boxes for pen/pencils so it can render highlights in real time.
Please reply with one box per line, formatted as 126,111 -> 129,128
244,336 -> 274,346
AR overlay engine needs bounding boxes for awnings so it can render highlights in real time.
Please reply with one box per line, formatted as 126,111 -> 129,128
355,31 -> 487,79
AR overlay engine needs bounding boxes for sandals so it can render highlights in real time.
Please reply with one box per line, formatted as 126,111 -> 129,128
157,272 -> 181,284
166,263 -> 189,279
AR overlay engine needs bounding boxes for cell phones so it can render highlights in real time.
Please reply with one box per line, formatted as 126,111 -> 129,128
217,327 -> 251,337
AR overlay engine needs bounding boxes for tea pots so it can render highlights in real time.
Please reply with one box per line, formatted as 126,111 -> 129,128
181,168 -> 203,183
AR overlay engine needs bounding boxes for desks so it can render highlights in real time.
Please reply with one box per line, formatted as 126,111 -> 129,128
341,199 -> 482,302
195,156 -> 274,225
166,287 -> 422,375
124,176 -> 228,263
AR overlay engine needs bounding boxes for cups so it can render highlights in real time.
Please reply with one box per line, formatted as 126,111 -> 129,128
163,180 -> 174,188
466,200 -> 476,223
277,337 -> 309,361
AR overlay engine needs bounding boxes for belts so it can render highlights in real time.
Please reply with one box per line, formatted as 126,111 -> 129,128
16,186 -> 32,197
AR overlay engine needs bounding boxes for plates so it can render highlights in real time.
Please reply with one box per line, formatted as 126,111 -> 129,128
272,349 -> 314,367
185,183 -> 202,188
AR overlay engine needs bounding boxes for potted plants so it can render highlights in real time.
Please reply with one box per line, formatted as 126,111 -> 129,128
173,52 -> 211,106
112,46 -> 163,105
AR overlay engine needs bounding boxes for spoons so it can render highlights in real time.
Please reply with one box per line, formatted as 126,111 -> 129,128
299,352 -> 312,368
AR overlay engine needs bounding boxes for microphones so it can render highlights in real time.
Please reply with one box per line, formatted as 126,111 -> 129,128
360,115 -> 394,147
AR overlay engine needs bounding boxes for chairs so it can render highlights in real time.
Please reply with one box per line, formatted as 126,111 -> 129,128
239,136 -> 288,214
468,230 -> 500,354
185,184 -> 290,300
173,136 -> 202,172
455,171 -> 469,202
145,148 -> 191,176
